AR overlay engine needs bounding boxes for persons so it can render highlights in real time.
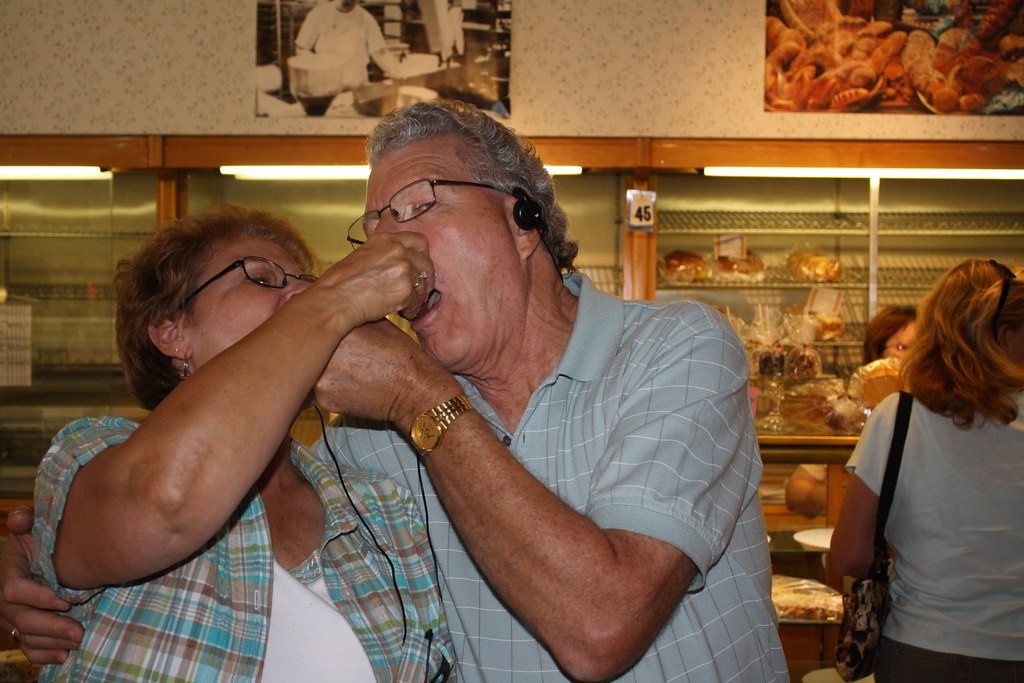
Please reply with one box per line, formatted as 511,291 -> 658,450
1,101 -> 791,682
294,0 -> 402,117
29,202 -> 459,682
784,258 -> 1024,683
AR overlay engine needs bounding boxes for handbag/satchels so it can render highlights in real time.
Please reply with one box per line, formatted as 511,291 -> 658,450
833,390 -> 913,683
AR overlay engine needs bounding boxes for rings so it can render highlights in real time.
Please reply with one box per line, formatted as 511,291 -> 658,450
9,630 -> 19,639
414,270 -> 429,289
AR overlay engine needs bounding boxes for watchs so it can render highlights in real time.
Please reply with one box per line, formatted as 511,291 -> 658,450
409,395 -> 475,458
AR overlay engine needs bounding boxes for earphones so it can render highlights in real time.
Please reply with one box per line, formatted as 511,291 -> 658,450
510,187 -> 543,231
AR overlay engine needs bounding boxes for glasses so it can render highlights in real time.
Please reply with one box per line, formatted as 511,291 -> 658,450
347,178 -> 513,251
884,344 -> 910,350
176,257 -> 319,310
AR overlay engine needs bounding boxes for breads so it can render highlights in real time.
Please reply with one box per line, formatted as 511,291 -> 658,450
661,247 -> 843,284
774,591 -> 844,618
723,309 -> 903,433
